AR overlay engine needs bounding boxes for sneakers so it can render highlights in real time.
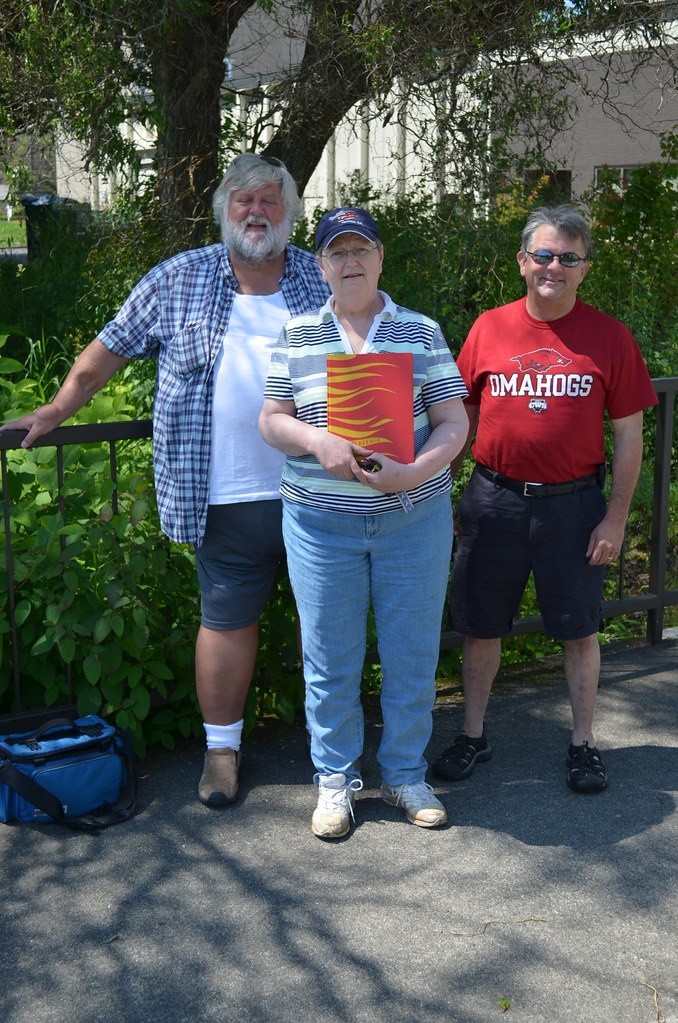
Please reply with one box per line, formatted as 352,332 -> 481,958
197,747 -> 243,807
565,743 -> 610,794
380,780 -> 452,830
430,729 -> 493,782
310,760 -> 364,838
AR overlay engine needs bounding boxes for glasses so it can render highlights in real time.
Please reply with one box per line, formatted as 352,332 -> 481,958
524,247 -> 587,268
321,244 -> 378,261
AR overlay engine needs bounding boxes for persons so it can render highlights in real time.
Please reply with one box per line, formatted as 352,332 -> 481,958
447,202 -> 661,798
255,206 -> 478,842
1,155 -> 337,808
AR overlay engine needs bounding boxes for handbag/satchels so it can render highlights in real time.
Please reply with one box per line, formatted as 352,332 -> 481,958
0,713 -> 139,827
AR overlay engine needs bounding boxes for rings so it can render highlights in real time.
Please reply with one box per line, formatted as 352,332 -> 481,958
608,556 -> 613,560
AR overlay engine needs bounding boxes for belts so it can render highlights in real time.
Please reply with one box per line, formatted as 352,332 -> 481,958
475,462 -> 600,500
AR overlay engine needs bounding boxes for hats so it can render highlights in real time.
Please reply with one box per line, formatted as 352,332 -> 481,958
314,207 -> 380,252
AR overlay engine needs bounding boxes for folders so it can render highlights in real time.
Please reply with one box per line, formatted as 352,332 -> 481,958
326,352 -> 415,465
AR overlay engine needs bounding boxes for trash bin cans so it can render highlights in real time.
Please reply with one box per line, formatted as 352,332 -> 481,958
19,196 -> 85,262
